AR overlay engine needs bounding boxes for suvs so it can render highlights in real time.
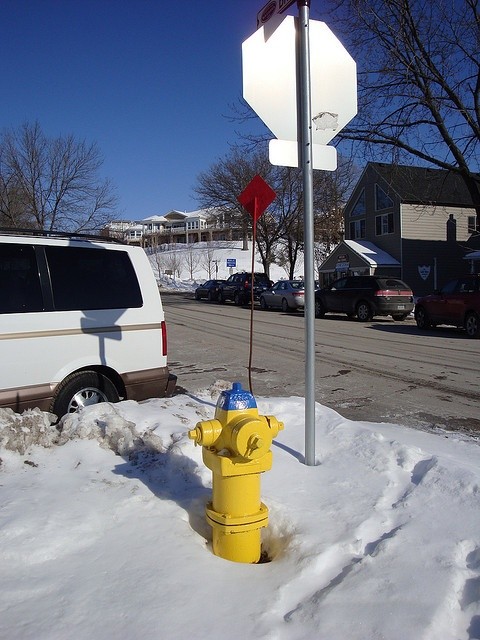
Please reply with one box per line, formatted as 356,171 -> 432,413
216,271 -> 272,306
0,228 -> 178,426
315,277 -> 414,320
414,273 -> 480,338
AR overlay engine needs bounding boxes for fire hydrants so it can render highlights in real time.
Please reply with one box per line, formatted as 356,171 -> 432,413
187,381 -> 285,564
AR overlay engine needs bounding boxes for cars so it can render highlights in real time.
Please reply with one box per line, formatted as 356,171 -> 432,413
259,280 -> 305,313
194,279 -> 227,301
